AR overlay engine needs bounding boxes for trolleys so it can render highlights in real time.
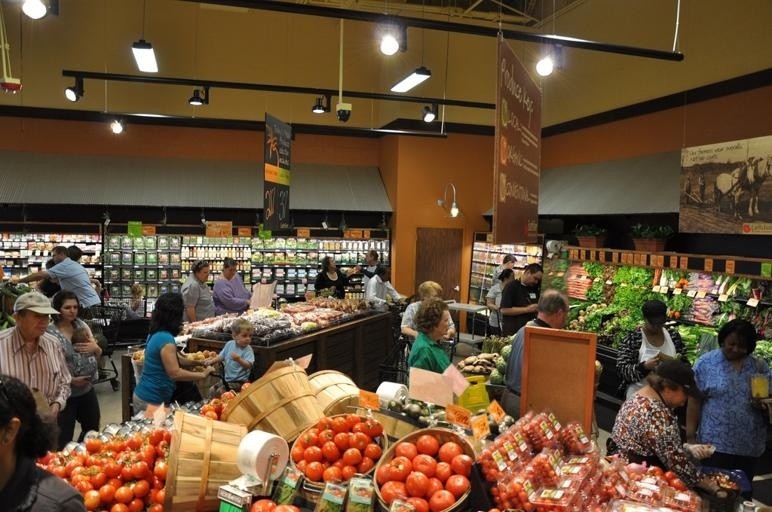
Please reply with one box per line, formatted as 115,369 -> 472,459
66,289 -> 124,394
96,284 -> 147,350
442,296 -> 489,358
378,325 -> 458,388
387,294 -> 418,340
202,358 -> 231,392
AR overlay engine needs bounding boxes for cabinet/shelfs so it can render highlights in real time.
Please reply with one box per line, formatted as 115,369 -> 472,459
104,222 -> 181,316
1,221 -> 103,306
252,239 -> 391,300
181,237 -> 252,299
468,241 -> 542,320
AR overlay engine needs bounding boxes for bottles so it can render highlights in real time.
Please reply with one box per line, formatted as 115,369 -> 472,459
181,246 -> 252,273
741,499 -> 762,512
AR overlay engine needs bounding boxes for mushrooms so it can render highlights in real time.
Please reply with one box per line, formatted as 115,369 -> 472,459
457,353 -> 495,374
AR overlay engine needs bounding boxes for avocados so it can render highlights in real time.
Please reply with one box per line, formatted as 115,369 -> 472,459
390,399 -> 428,427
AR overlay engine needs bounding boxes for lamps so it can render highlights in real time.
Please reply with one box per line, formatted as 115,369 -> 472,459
130,1 -> 160,74
21,0 -> 60,21
437,183 -> 460,218
389,0 -> 433,94
378,27 -> 563,78
61,75 -> 439,125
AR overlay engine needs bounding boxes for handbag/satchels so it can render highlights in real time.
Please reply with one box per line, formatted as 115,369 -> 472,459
171,380 -> 202,405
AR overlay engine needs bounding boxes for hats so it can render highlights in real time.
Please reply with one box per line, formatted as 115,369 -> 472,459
655,359 -> 704,400
13,290 -> 61,315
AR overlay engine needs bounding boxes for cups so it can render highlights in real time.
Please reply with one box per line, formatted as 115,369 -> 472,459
750,372 -> 770,400
345,292 -> 365,300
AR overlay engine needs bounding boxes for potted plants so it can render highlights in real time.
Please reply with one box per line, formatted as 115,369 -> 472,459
629,222 -> 674,252
575,223 -> 610,248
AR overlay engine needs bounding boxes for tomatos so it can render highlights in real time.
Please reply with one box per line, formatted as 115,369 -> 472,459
251,500 -> 298,512
377,436 -> 473,511
294,412 -> 380,484
37,428 -> 171,511
201,381 -> 252,418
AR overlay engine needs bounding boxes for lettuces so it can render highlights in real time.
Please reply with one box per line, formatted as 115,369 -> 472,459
612,266 -> 650,311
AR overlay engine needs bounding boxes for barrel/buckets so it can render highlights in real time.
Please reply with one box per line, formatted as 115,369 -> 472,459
373,427 -> 478,512
434,422 -> 497,462
370,408 -> 427,449
288,413 -> 391,511
184,362 -> 222,400
161,409 -> 249,512
220,365 -> 327,444
308,369 -> 361,417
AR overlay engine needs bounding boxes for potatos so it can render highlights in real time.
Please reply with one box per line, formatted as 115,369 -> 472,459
186,350 -> 216,360
569,303 -> 606,328
133,350 -> 145,359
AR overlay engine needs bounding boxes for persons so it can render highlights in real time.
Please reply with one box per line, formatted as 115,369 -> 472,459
314,256 -> 354,299
180,259 -> 216,323
356,249 -> 381,300
0,373 -> 87,510
0,245 -> 106,451
365,266 -> 405,307
400,280 -> 457,364
485,252 -> 544,337
201,319 -> 256,402
124,283 -> 145,320
212,257 -> 251,316
697,173 -> 707,202
502,287 -> 570,421
607,354 -> 716,490
684,174 -> 694,202
132,292 -> 216,416
617,301 -> 690,403
685,319 -> 771,501
407,297 -> 453,387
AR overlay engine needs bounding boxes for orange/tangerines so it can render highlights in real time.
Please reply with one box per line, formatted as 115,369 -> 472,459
477,410 -> 699,511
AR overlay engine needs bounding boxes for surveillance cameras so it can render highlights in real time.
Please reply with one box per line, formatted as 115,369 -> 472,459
336,103 -> 352,121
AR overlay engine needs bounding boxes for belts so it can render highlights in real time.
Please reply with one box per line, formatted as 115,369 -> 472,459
507,387 -> 520,397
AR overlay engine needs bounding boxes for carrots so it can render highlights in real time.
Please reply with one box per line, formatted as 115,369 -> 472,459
565,264 -> 591,300
